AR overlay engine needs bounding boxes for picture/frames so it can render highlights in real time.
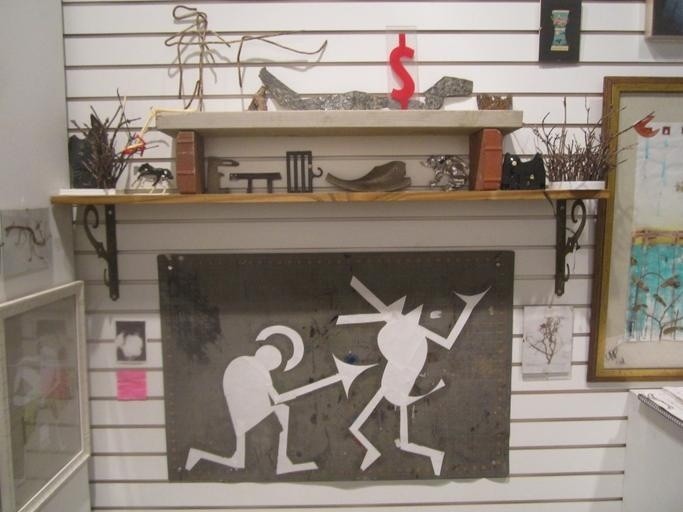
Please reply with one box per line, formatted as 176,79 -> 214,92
0,279 -> 92,512
584,73 -> 683,384
536,0 -> 583,64
642,0 -> 683,44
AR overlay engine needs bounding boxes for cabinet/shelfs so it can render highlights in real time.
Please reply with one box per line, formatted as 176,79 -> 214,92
50,106 -> 612,301
